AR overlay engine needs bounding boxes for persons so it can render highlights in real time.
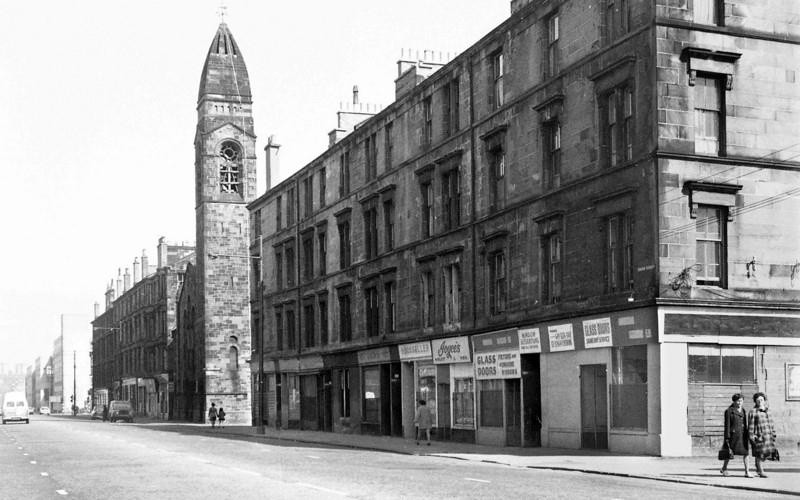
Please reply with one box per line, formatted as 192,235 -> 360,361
748,392 -> 777,478
720,393 -> 754,478
217,407 -> 225,428
208,402 -> 218,428
413,400 -> 432,446
102,404 -> 109,422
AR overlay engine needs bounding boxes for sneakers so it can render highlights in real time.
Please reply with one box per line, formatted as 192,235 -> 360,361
745,472 -> 752,478
720,468 -> 729,477
760,473 -> 767,477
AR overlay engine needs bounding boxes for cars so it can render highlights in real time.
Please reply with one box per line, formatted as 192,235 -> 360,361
28,407 -> 34,413
40,407 -> 50,415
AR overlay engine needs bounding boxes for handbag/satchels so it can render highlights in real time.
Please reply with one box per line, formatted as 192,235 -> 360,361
767,449 -> 780,461
718,440 -> 734,459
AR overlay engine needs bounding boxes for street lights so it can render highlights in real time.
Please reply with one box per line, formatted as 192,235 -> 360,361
257,280 -> 264,433
74,350 -> 77,416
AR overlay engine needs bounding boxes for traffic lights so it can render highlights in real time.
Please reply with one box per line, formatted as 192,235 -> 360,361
71,395 -> 73,402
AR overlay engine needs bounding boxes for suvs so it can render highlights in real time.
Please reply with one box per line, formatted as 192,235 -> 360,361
109,400 -> 133,422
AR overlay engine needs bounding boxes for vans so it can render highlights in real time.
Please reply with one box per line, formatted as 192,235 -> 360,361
2,391 -> 30,423
